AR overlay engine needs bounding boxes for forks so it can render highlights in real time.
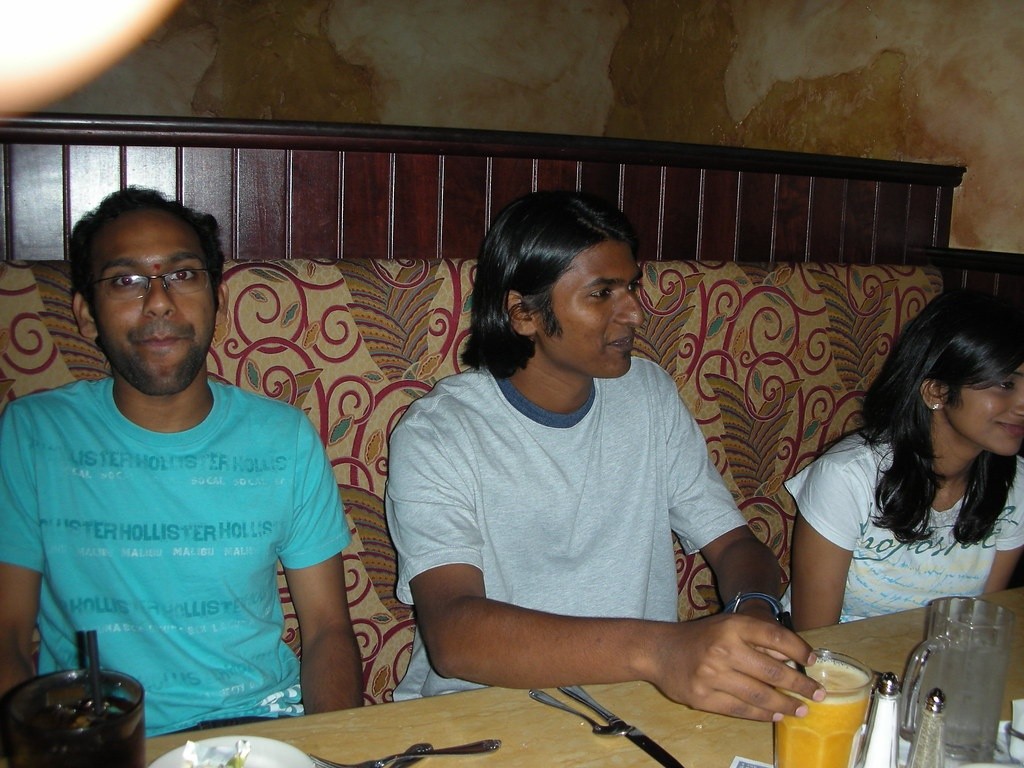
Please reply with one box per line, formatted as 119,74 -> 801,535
307,738 -> 505,768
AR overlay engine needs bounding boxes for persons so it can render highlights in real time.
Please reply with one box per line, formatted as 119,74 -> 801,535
781,290 -> 1024,633
384,191 -> 827,722
0,186 -> 366,739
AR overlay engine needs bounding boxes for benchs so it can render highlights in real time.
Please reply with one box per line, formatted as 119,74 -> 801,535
0,259 -> 946,706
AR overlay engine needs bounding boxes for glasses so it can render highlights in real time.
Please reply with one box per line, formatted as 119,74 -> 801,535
89,268 -> 210,301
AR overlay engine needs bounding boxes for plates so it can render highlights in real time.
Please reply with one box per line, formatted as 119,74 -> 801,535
147,735 -> 317,768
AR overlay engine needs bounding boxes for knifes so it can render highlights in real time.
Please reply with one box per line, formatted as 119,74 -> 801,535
558,684 -> 685,768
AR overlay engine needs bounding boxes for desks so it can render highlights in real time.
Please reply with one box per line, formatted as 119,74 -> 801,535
141,587 -> 1024,768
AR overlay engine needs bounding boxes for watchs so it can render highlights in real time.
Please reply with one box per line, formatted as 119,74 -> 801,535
723,594 -> 795,635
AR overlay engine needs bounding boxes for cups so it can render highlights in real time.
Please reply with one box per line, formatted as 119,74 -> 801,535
773,646 -> 877,767
0,668 -> 147,767
897,596 -> 1015,762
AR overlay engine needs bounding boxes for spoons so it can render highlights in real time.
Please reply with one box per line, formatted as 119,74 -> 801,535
528,690 -> 636,736
